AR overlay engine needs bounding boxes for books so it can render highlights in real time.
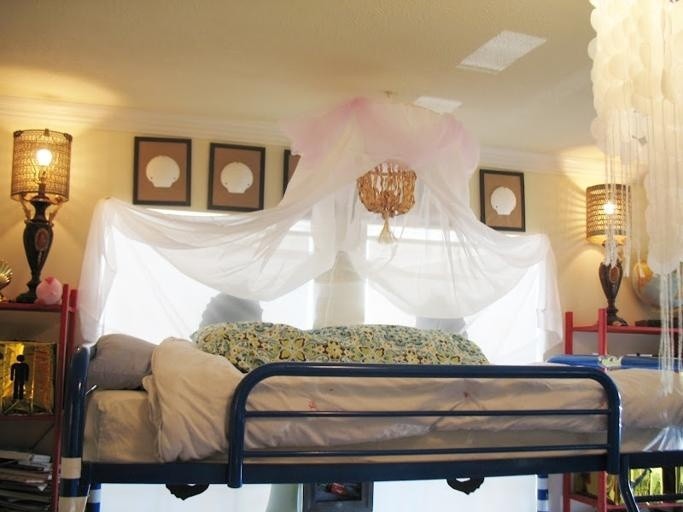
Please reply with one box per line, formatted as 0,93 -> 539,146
0,449 -> 54,512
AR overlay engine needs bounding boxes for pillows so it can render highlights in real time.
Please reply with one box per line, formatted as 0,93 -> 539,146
83,333 -> 158,391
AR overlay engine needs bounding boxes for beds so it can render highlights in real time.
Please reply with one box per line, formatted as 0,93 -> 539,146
72,363 -> 683,512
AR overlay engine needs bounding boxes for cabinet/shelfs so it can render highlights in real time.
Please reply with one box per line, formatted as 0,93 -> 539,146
0,281 -> 79,512
563,308 -> 683,512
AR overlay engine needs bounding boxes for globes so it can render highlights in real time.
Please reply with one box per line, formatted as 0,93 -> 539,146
632,257 -> 683,327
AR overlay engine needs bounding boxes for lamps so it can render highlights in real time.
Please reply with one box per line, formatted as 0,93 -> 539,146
585,183 -> 634,327
8,127 -> 72,303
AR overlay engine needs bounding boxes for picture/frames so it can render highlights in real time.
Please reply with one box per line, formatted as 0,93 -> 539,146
206,141 -> 266,211
303,475 -> 373,512
283,148 -> 302,195
131,135 -> 193,206
479,168 -> 526,232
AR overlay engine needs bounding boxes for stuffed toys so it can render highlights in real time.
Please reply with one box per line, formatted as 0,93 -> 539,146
35,276 -> 64,306
